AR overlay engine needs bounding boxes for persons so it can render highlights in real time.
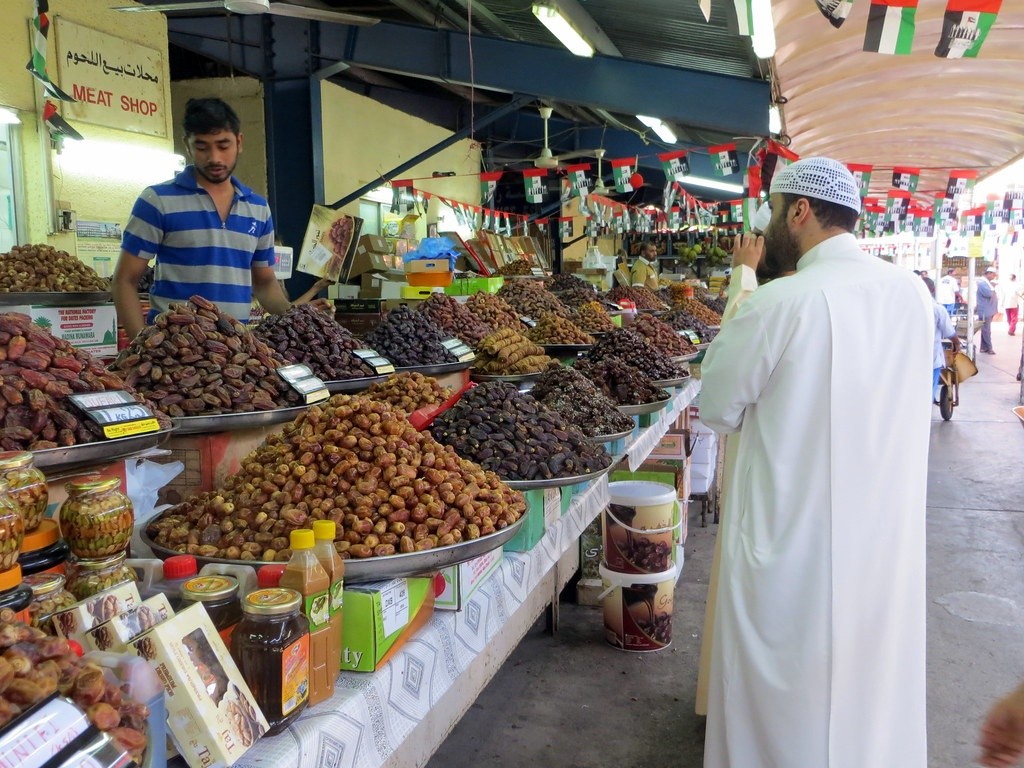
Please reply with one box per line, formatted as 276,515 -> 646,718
1000,274 -> 1024,336
976,266 -> 998,355
695,157 -> 935,768
631,240 -> 660,293
912,268 -> 961,405
110,96 -> 337,346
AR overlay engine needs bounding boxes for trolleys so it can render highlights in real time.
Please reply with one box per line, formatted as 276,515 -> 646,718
954,318 -> 985,346
954,297 -> 968,316
936,348 -> 979,421
1011,404 -> 1024,428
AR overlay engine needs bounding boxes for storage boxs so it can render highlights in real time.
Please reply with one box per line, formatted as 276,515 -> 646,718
575,392 -> 731,604
1,233 -> 550,767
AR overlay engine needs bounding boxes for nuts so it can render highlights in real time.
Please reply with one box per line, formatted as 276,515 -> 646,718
85,596 -> 266,747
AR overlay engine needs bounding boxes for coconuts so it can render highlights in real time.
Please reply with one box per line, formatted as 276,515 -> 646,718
679,244 -> 728,268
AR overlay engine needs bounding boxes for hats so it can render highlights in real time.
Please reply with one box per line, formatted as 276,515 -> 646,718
986,267 -> 996,273
770,157 -> 862,215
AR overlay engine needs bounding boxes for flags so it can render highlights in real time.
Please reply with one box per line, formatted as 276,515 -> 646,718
24,0 -> 85,143
934,0 -> 1003,59
564,163 -> 595,196
388,135 -> 1024,256
610,154 -> 641,193
522,167 -> 550,204
814,0 -> 853,29
863,0 -> 918,55
477,170 -> 505,205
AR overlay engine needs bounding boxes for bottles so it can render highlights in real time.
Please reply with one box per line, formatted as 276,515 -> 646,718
177,576 -> 240,655
236,588 -> 310,737
582,246 -> 608,291
312,520 -> 345,681
278,528 -> 333,707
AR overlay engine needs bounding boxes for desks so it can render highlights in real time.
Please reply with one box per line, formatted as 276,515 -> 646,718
227,373 -> 705,768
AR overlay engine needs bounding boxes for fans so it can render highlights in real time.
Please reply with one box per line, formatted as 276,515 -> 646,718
483,106 -> 598,169
551,150 -> 653,198
111,0 -> 381,29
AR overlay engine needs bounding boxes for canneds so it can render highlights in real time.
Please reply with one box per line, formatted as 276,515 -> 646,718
0,451 -> 139,626
178,576 -> 309,738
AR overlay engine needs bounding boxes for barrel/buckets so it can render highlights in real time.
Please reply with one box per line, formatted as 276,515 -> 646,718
596,559 -> 677,653
126,556 -> 198,612
67,639 -> 167,768
198,563 -> 286,601
600,480 -> 682,574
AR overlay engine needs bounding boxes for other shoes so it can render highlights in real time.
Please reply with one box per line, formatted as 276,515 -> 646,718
980,348 -> 996,354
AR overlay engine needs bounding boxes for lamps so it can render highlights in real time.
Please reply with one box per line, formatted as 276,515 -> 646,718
0,105 -> 22,124
56,134 -> 184,189
768,106 -> 783,135
750,1 -> 778,59
529,1 -> 596,58
637,114 -> 678,146
682,175 -> 749,196
